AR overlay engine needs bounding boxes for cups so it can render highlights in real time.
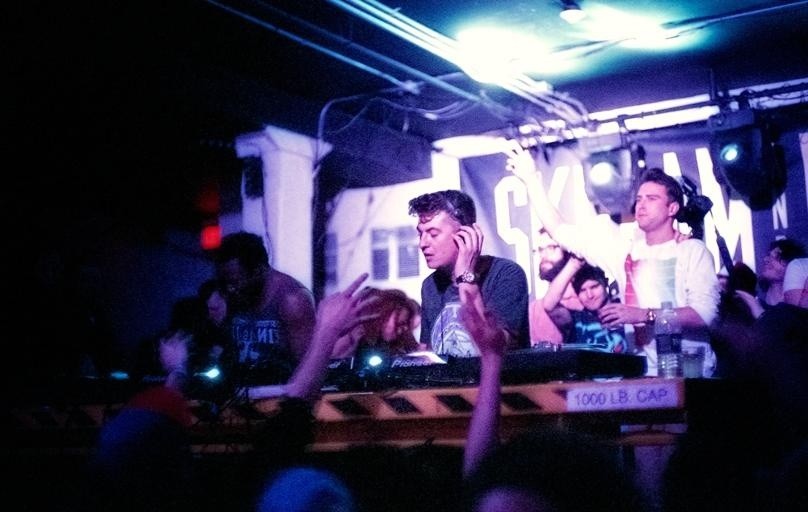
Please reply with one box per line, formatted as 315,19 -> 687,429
679,344 -> 706,378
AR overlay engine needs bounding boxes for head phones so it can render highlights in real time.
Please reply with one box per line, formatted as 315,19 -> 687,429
432,191 -> 478,244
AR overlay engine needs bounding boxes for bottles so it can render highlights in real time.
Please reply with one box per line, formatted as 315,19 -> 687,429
653,299 -> 686,379
